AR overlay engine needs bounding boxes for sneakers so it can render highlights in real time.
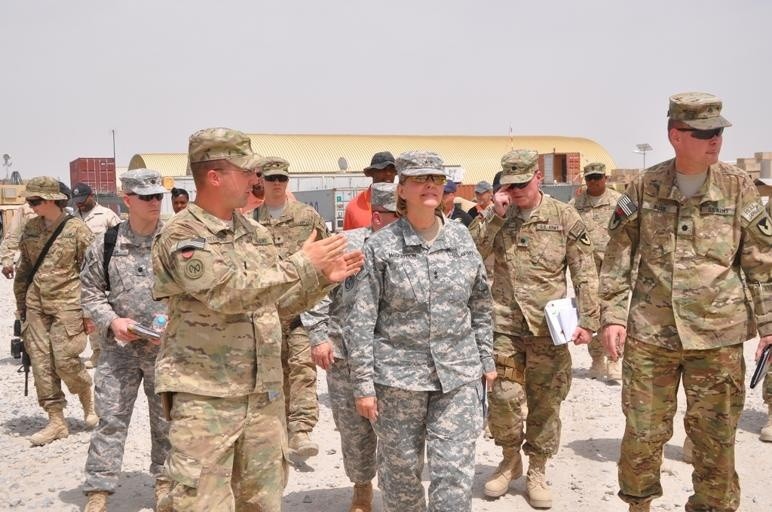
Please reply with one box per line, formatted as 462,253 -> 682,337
606,359 -> 622,383
289,429 -> 320,466
83,348 -> 101,370
82,489 -> 110,512
616,490 -> 664,512
154,478 -> 171,508
348,481 -> 374,512
589,357 -> 605,377
682,434 -> 696,466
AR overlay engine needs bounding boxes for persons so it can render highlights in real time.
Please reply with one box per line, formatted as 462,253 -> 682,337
243,155 -> 333,465
79,166 -> 185,511
341,145 -> 501,512
756,196 -> 772,442
466,148 -> 606,511
1,162 -> 633,447
342,147 -> 398,233
295,180 -> 403,512
596,89 -> 771,512
150,125 -> 366,511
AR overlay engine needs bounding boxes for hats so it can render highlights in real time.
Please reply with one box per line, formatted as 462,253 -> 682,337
474,181 -> 492,194
118,167 -> 170,196
370,181 -> 398,213
666,91 -> 733,132
492,170 -> 504,194
499,149 -> 539,185
363,151 -> 398,177
443,179 -> 456,193
260,156 -> 290,177
17,175 -> 69,201
582,162 -> 606,178
71,183 -> 92,206
188,127 -> 272,171
394,150 -> 450,184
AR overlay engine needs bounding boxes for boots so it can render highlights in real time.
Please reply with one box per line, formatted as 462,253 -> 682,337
77,385 -> 100,428
481,444 -> 523,498
27,404 -> 70,447
525,450 -> 553,509
759,402 -> 772,443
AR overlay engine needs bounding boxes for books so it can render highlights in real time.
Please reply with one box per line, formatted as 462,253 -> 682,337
749,347 -> 771,388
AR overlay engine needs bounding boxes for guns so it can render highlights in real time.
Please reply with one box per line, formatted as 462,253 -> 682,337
11,320 -> 31,396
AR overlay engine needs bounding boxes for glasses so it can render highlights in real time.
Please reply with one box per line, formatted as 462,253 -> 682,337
126,192 -> 164,202
263,175 -> 288,183
26,198 -> 45,207
406,174 -> 447,186
675,126 -> 725,141
506,180 -> 531,190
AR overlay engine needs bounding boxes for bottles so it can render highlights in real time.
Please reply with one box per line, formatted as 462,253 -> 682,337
147,315 -> 167,334
